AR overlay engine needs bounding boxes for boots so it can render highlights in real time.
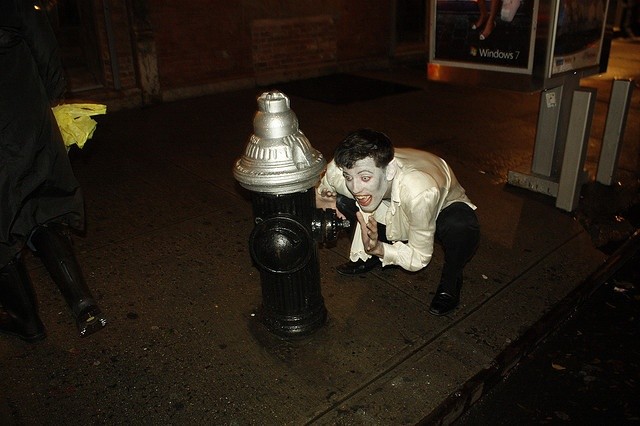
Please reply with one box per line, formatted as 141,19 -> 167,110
1,258 -> 47,341
31,221 -> 108,339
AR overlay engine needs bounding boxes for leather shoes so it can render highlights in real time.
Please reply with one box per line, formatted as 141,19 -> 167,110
336,255 -> 382,277
429,270 -> 463,316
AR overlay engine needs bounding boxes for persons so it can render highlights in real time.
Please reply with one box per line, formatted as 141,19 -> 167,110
0,1 -> 108,344
471,1 -> 500,42
315,125 -> 481,317
